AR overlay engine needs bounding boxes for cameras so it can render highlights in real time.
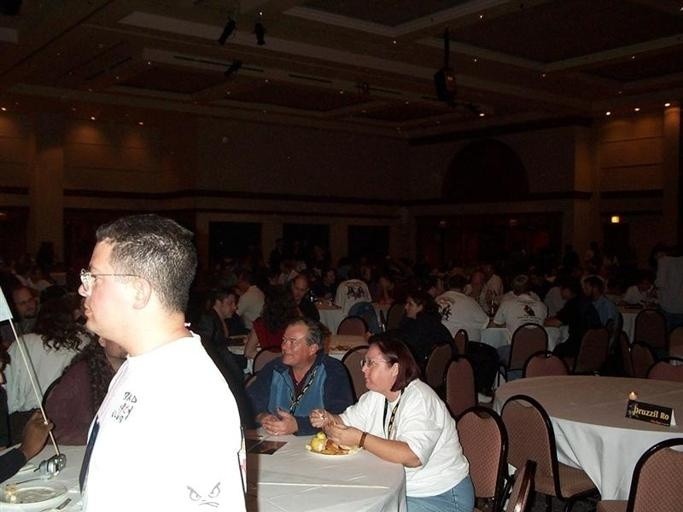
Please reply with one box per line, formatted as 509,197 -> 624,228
40,453 -> 67,474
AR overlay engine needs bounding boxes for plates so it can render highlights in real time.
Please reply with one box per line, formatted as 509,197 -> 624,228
0,479 -> 68,512
306,445 -> 347,458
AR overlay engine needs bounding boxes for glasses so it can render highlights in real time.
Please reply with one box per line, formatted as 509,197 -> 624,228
80,268 -> 144,293
360,357 -> 390,369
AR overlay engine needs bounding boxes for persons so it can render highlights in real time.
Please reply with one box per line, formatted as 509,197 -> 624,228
0,240 -> 682,511
77,215 -> 251,511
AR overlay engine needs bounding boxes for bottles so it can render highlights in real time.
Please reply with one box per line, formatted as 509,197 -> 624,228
310,290 -> 314,303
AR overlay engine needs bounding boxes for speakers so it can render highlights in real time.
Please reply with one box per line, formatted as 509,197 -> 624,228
434,66 -> 457,103
